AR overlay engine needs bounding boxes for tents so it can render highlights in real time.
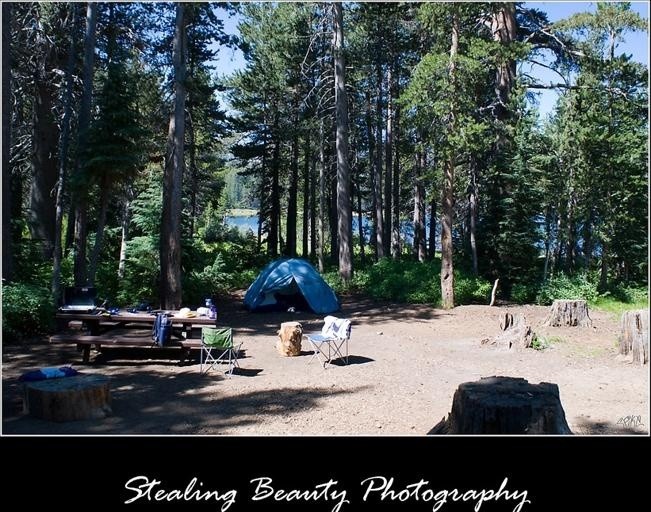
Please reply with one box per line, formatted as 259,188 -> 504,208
240,254 -> 342,316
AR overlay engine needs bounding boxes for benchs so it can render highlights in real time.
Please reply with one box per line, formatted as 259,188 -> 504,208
67,321 -> 215,355
51,334 -> 215,366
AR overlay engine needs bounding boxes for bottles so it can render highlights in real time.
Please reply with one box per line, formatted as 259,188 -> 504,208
209,304 -> 217,319
205,298 -> 214,308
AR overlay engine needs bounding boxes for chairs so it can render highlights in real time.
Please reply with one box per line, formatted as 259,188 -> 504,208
200,326 -> 243,377
308,315 -> 353,370
